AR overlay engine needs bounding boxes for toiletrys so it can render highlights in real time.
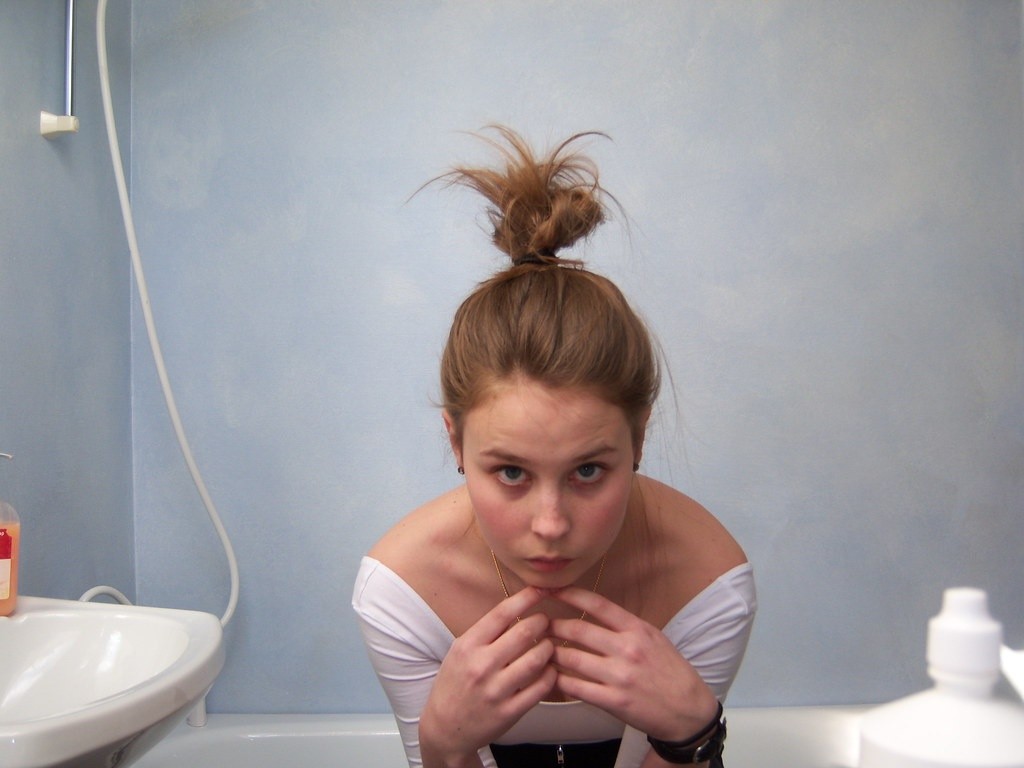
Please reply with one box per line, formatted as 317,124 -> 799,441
856,583 -> 1023,765
0,448 -> 20,618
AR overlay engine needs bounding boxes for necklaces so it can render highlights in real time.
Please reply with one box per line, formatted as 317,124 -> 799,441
490,542 -> 607,665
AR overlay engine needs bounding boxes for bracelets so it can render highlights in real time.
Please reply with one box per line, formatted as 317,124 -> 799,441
647,701 -> 727,764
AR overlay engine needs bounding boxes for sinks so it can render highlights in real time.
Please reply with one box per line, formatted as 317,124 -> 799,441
0,595 -> 223,767
108,706 -> 1023,766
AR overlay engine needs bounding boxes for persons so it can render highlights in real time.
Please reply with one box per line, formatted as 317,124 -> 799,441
350,127 -> 756,768
